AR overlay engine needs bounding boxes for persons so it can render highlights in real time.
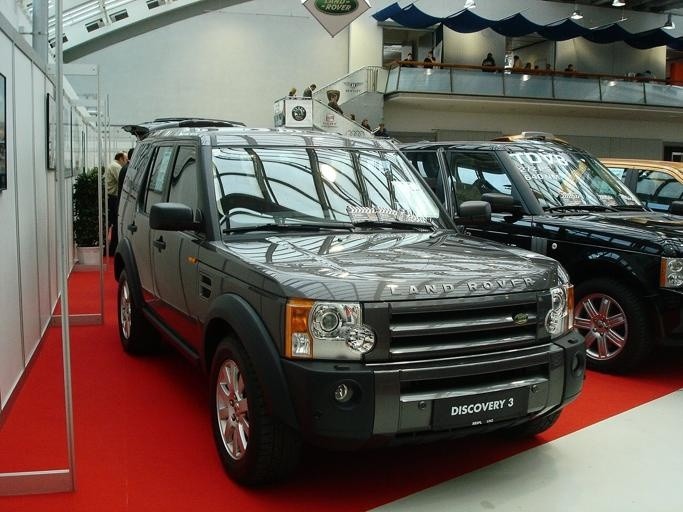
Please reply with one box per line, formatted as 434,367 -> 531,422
422,51 -> 435,68
290,82 -> 357,119
509,51 -> 583,76
480,51 -> 500,73
378,123 -> 389,134
402,51 -> 416,62
103,150 -> 129,227
362,117 -> 371,130
108,148 -> 137,256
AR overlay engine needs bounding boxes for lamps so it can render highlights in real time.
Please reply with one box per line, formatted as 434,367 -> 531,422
463,0 -> 677,30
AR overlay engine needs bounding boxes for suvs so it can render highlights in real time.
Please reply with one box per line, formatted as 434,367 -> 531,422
490,132 -> 682,216
393,131 -> 682,372
110,116 -> 586,489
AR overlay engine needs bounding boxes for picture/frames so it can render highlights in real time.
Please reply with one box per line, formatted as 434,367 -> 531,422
45,91 -> 56,170
0,72 -> 7,191
